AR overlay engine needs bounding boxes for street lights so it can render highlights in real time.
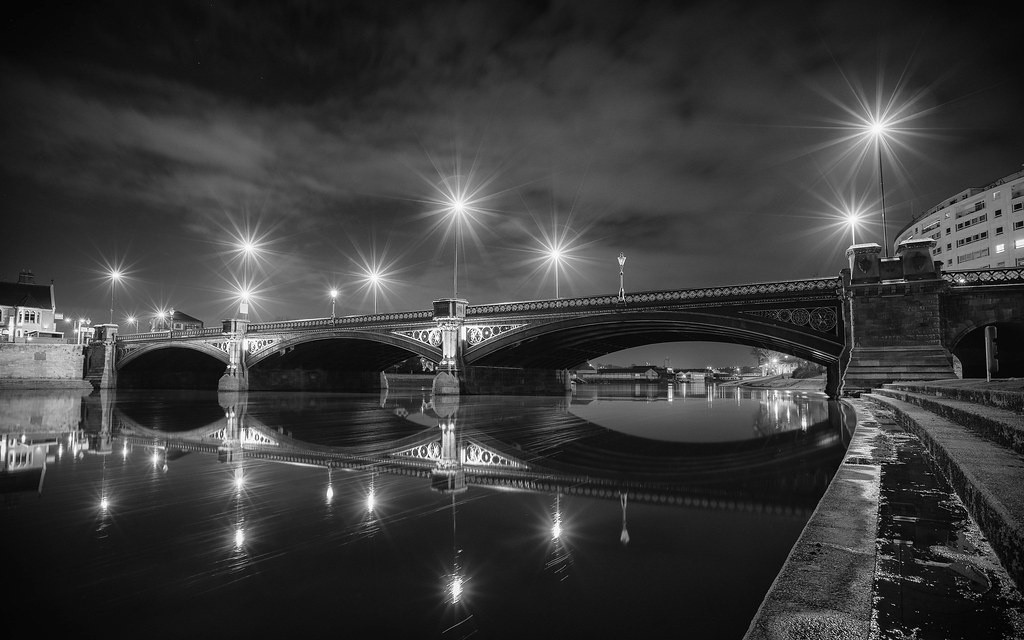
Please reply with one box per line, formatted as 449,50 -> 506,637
617,251 -> 627,293
85,319 -> 91,344
849,214 -> 857,246
78,318 -> 85,344
330,289 -> 338,319
110,271 -> 119,324
553,250 -> 561,299
371,272 -> 380,315
454,202 -> 464,299
871,120 -> 889,258
169,306 -> 175,339
